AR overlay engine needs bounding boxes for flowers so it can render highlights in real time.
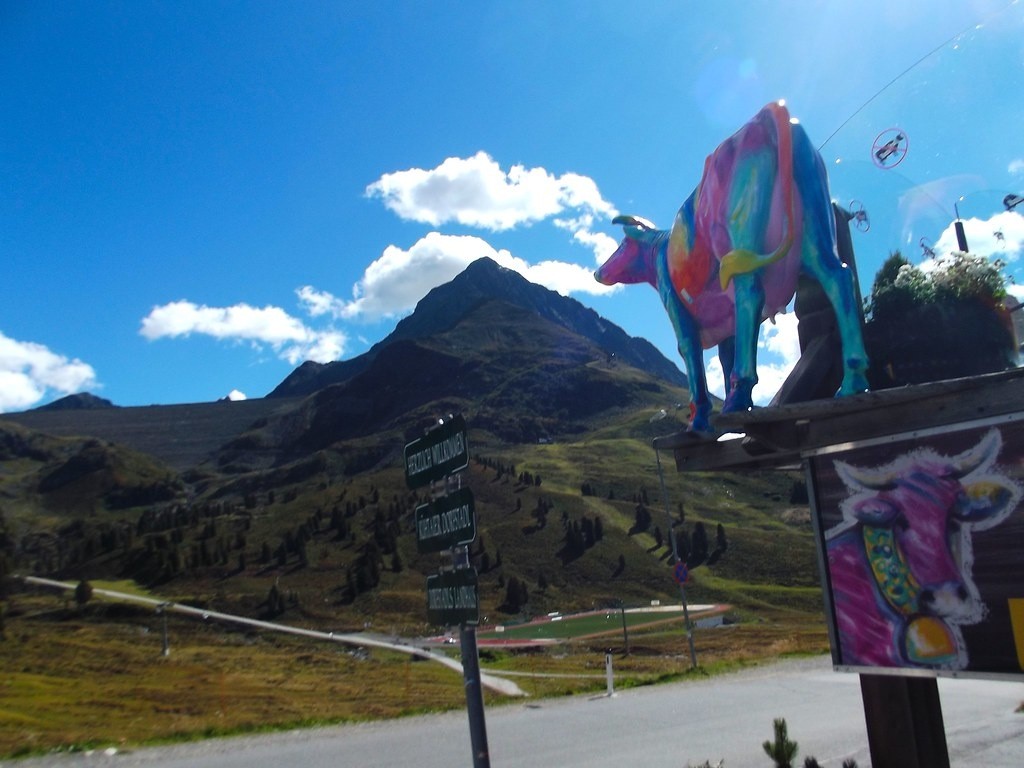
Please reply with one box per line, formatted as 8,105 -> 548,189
863,249 -> 1016,323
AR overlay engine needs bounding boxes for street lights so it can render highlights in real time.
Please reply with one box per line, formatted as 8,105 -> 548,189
650,431 -> 747,669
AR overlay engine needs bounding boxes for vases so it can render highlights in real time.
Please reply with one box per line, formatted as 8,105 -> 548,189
859,291 -> 1019,388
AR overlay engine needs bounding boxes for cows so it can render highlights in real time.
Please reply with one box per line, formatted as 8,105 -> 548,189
592,102 -> 872,435
